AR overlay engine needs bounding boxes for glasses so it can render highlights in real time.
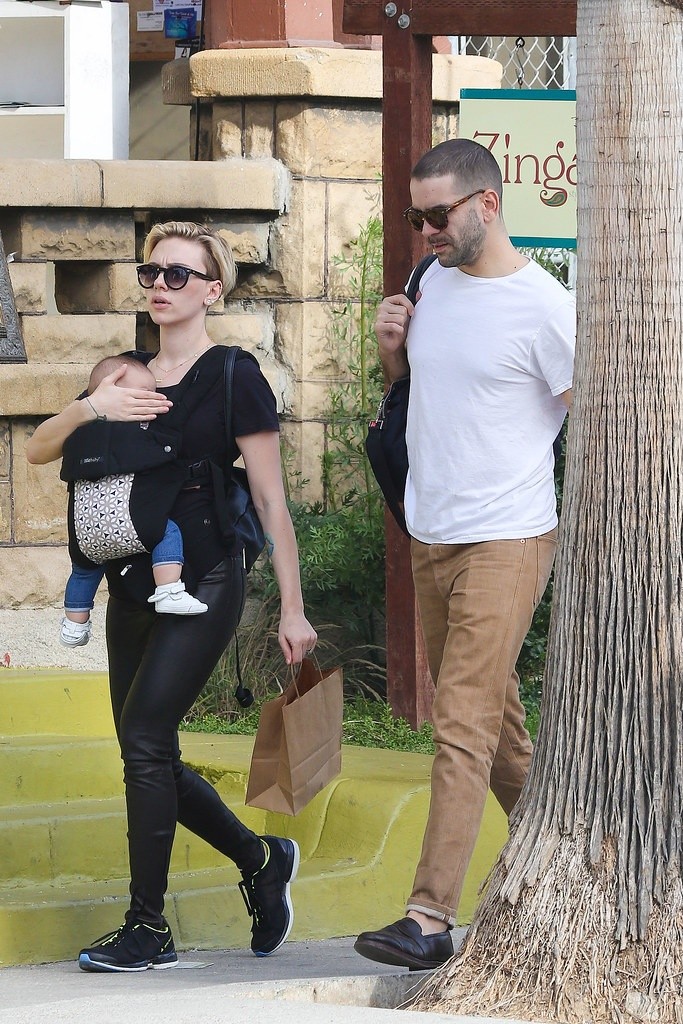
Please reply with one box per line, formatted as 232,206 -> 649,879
136,265 -> 214,290
404,189 -> 484,231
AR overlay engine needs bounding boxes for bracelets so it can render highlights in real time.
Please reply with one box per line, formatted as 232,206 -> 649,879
85,396 -> 108,422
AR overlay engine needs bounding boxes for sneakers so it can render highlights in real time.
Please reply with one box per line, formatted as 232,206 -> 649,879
238,837 -> 300,956
79,923 -> 178,973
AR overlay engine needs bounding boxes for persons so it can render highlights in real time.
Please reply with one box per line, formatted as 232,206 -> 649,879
351,140 -> 577,970
27,219 -> 320,973
57,354 -> 209,650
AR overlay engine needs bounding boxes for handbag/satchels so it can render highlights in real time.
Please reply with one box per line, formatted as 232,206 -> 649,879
365,252 -> 441,540
225,345 -> 266,574
242,649 -> 344,815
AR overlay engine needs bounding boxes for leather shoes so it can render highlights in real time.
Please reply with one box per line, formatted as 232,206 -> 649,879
354,917 -> 454,970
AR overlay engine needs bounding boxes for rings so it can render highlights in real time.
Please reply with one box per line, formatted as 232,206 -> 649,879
306,647 -> 312,652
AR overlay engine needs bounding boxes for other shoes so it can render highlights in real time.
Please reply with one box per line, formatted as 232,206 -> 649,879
147,583 -> 207,615
60,617 -> 90,647
